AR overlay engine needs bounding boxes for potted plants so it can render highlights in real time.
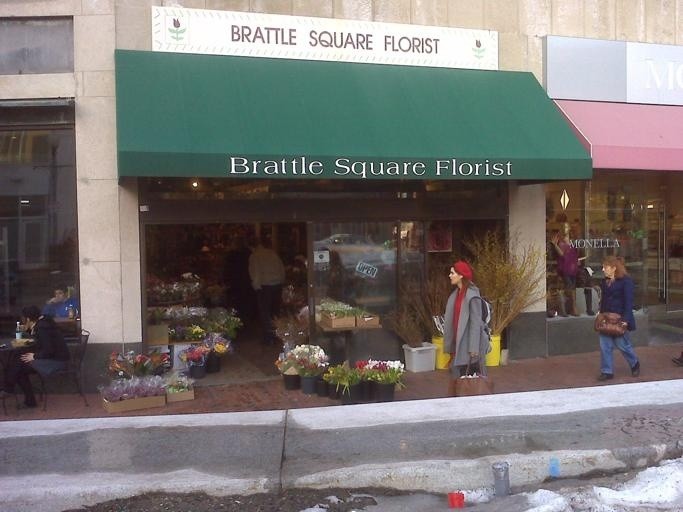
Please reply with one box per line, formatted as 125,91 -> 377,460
459,227 -> 558,367
319,294 -> 380,328
384,304 -> 436,374
400,266 -> 458,370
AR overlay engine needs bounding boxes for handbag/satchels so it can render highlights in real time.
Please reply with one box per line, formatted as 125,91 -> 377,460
595,281 -> 626,336
455,355 -> 493,397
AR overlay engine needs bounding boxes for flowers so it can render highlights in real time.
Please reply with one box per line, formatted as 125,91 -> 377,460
273,343 -> 301,375
316,353 -> 330,380
101,375 -> 164,403
167,374 -> 190,397
286,344 -> 329,377
333,361 -> 360,400
36,328 -> 91,412
147,306 -> 242,340
178,344 -> 209,367
357,361 -> 374,383
202,335 -> 235,358
104,351 -> 172,377
367,360 -> 406,389
323,366 -> 339,384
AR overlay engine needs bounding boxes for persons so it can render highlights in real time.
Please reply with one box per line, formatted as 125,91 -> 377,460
442,261 -> 493,397
594,255 -> 641,381
246,238 -> 287,345
550,221 -> 588,318
42,285 -> 77,317
0,305 -> 71,410
671,350 -> 683,367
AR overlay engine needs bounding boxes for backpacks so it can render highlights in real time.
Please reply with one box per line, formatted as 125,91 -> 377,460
468,296 -> 493,342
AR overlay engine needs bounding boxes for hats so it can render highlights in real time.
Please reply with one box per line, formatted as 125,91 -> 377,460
454,262 -> 473,280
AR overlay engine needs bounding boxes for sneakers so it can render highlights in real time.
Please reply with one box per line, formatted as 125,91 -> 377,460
598,373 -> 612,381
0,390 -> 14,399
671,353 -> 683,365
17,402 -> 37,410
632,362 -> 640,376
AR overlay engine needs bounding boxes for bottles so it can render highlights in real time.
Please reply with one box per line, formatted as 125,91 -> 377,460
16,322 -> 22,340
69,305 -> 74,320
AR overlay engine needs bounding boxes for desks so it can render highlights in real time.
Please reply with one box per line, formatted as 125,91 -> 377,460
52,316 -> 81,335
0,336 -> 41,416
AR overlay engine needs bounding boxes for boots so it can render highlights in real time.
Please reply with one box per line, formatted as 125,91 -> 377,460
567,290 -> 579,316
584,289 -> 595,316
558,296 -> 568,317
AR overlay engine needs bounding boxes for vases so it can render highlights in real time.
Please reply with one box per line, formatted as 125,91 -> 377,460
329,382 -> 339,400
368,380 -> 378,399
204,350 -> 225,373
189,362 -> 205,379
302,374 -> 318,393
339,382 -> 362,404
318,382 -> 329,398
374,383 -> 398,403
282,373 -> 301,389
